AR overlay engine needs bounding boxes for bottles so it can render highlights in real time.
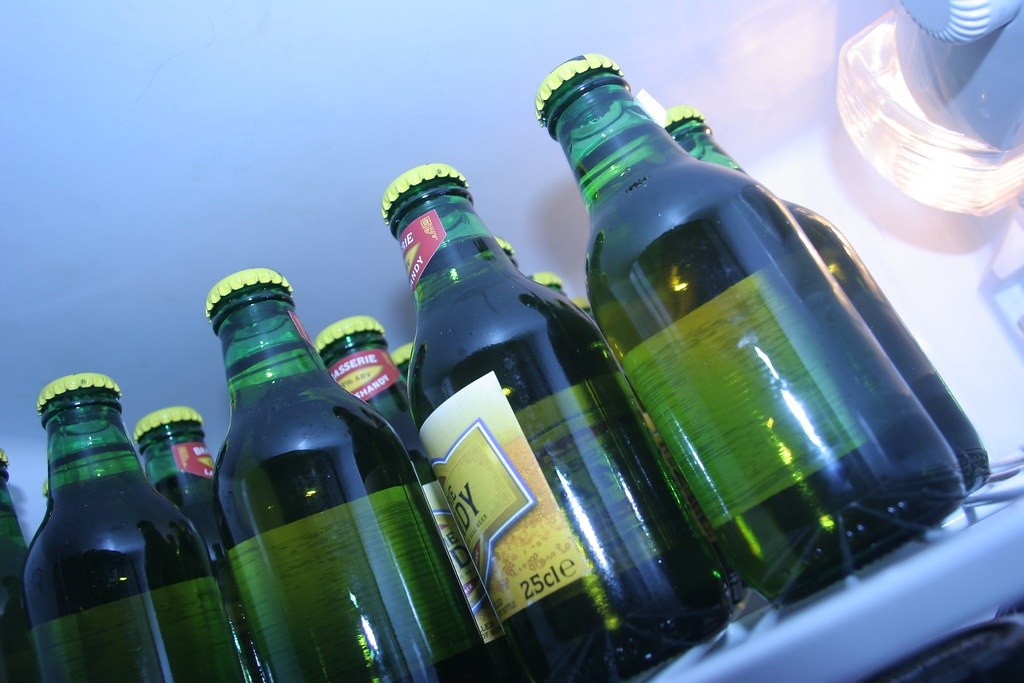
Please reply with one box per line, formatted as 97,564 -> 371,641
0,52 -> 992,683
